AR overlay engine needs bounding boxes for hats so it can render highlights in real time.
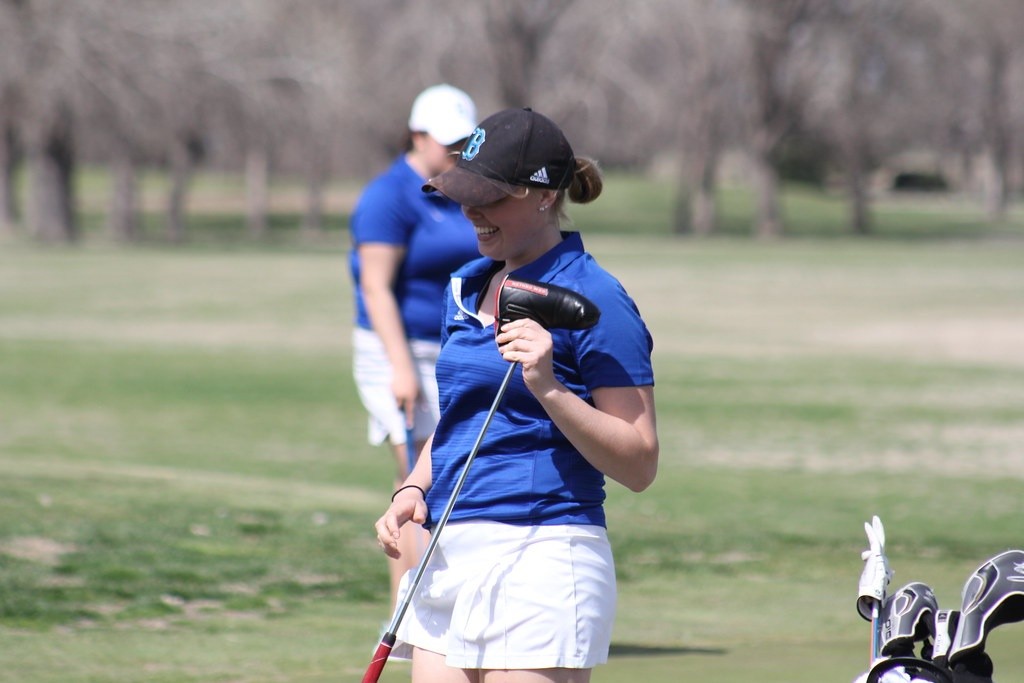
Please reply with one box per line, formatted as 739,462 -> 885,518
408,82 -> 477,147
422,106 -> 578,208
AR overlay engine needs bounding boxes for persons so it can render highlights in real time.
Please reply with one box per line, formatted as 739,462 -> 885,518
349,83 -> 486,662
374,107 -> 661,683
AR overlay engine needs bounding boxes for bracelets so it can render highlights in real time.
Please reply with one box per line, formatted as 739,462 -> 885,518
391,485 -> 426,502
